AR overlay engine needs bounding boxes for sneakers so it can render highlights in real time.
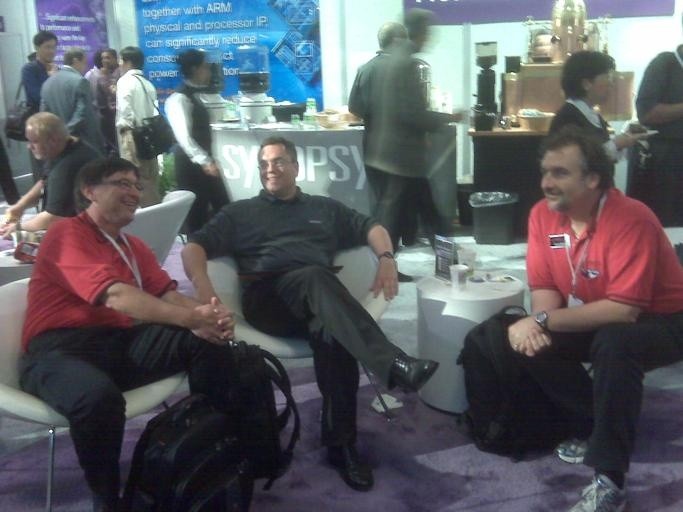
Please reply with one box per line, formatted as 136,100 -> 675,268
570,478 -> 627,512
555,433 -> 594,465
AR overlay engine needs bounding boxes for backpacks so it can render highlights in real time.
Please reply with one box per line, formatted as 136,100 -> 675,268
124,392 -> 256,507
185,338 -> 303,492
454,305 -> 597,465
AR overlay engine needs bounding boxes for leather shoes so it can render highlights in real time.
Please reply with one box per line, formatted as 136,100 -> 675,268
385,352 -> 440,393
324,440 -> 374,492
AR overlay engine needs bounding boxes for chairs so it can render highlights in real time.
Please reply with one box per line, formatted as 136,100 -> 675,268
0,276 -> 189,511
119,189 -> 197,270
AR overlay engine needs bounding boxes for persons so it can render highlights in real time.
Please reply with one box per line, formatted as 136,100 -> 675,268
163,48 -> 230,234
546,50 -> 650,184
1,111 -> 104,241
18,156 -> 238,507
20,29 -> 59,112
99,47 -> 121,110
624,42 -> 682,224
181,135 -> 440,492
361,7 -> 465,254
83,53 -> 104,104
506,122 -> 683,512
348,20 -> 423,248
114,45 -> 160,208
39,48 -> 98,146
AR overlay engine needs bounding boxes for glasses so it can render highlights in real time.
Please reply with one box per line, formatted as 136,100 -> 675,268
256,159 -> 298,169
99,178 -> 146,192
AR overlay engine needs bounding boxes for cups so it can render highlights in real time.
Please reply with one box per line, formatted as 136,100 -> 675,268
450,264 -> 468,294
457,249 -> 475,275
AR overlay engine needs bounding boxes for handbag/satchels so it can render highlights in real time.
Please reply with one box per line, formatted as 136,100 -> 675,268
2,73 -> 34,138
126,80 -> 176,160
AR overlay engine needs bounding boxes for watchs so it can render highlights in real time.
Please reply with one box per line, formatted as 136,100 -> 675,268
532,310 -> 549,333
533,309 -> 555,333
